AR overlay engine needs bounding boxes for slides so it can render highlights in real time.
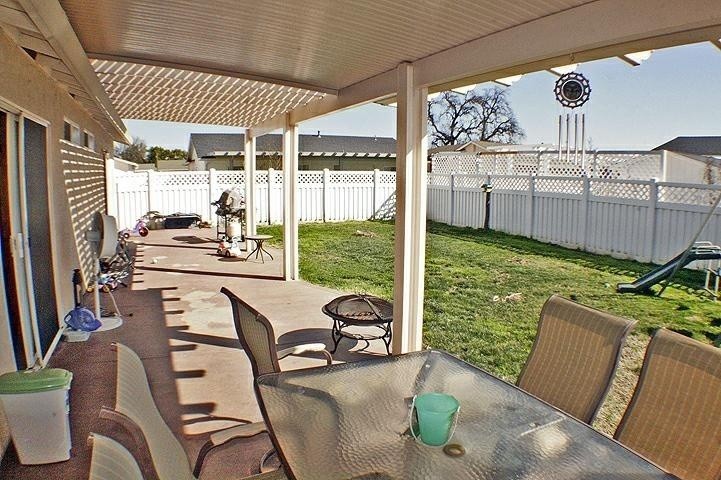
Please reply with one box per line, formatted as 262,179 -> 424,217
618,249 -> 695,293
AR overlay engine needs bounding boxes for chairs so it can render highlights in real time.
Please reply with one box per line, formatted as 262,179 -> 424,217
98,341 -> 290,479
84,432 -> 144,479
574,327 -> 721,480
219,286 -> 344,450
401,294 -> 641,480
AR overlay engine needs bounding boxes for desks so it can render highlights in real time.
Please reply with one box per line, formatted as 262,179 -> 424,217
243,233 -> 275,264
249,347 -> 682,480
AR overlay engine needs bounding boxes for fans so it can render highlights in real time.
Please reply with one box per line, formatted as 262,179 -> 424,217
83,211 -> 123,333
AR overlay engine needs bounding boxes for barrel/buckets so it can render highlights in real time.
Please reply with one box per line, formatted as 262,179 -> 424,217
409,393 -> 461,449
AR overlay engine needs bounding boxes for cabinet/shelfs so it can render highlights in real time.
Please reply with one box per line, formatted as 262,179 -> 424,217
217,211 -> 244,242
